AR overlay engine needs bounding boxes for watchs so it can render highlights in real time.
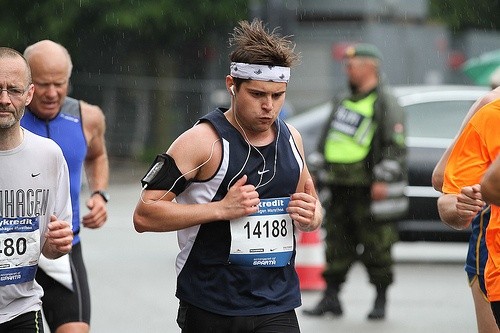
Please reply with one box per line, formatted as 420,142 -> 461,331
90,190 -> 110,203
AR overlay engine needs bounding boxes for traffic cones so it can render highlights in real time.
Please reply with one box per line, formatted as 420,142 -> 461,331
295,228 -> 328,292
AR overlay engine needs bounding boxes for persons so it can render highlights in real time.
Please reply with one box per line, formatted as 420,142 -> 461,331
133,17 -> 324,333
0,47 -> 74,333
431,68 -> 500,333
302,42 -> 409,318
20,39 -> 110,333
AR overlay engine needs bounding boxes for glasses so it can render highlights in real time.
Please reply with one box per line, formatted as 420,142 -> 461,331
0,82 -> 30,97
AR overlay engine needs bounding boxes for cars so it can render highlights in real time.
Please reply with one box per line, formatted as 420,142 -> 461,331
283,84 -> 494,244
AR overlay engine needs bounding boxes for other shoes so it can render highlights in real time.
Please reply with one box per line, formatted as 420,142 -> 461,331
369,296 -> 385,319
303,295 -> 341,315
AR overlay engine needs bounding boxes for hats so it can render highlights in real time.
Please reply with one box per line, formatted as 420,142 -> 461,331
345,42 -> 384,61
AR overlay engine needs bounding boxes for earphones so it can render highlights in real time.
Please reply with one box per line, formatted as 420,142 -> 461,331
230,85 -> 236,96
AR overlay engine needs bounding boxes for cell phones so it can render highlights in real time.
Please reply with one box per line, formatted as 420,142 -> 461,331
143,160 -> 164,183
